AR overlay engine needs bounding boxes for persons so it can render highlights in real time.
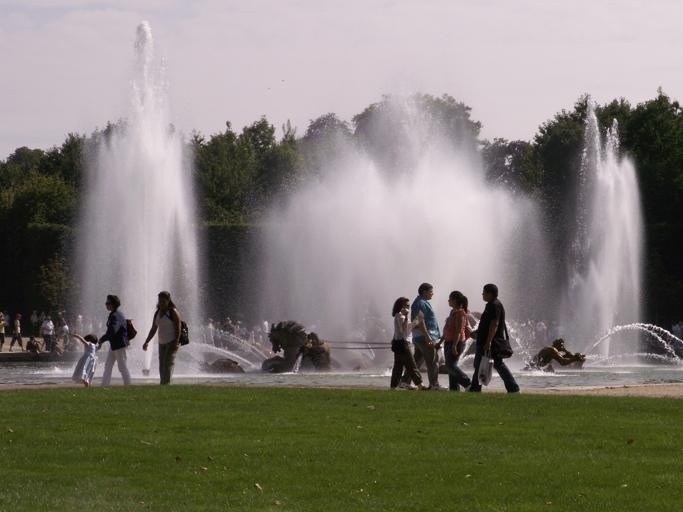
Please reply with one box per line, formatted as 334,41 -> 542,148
522,338 -> 585,371
142,290 -> 181,384
202,313 -> 275,364
504,316 -> 557,355
388,296 -> 432,392
94,294 -> 132,387
69,332 -> 101,388
465,283 -> 520,393
0,309 -> 100,355
397,282 -> 449,392
435,290 -> 472,392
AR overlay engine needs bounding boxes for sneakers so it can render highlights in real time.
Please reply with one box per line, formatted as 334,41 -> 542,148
391,384 -> 449,391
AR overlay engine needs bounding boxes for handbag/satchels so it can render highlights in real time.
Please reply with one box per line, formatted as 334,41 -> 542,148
478,350 -> 494,385
494,321 -> 513,358
179,321 -> 189,345
126,318 -> 137,341
